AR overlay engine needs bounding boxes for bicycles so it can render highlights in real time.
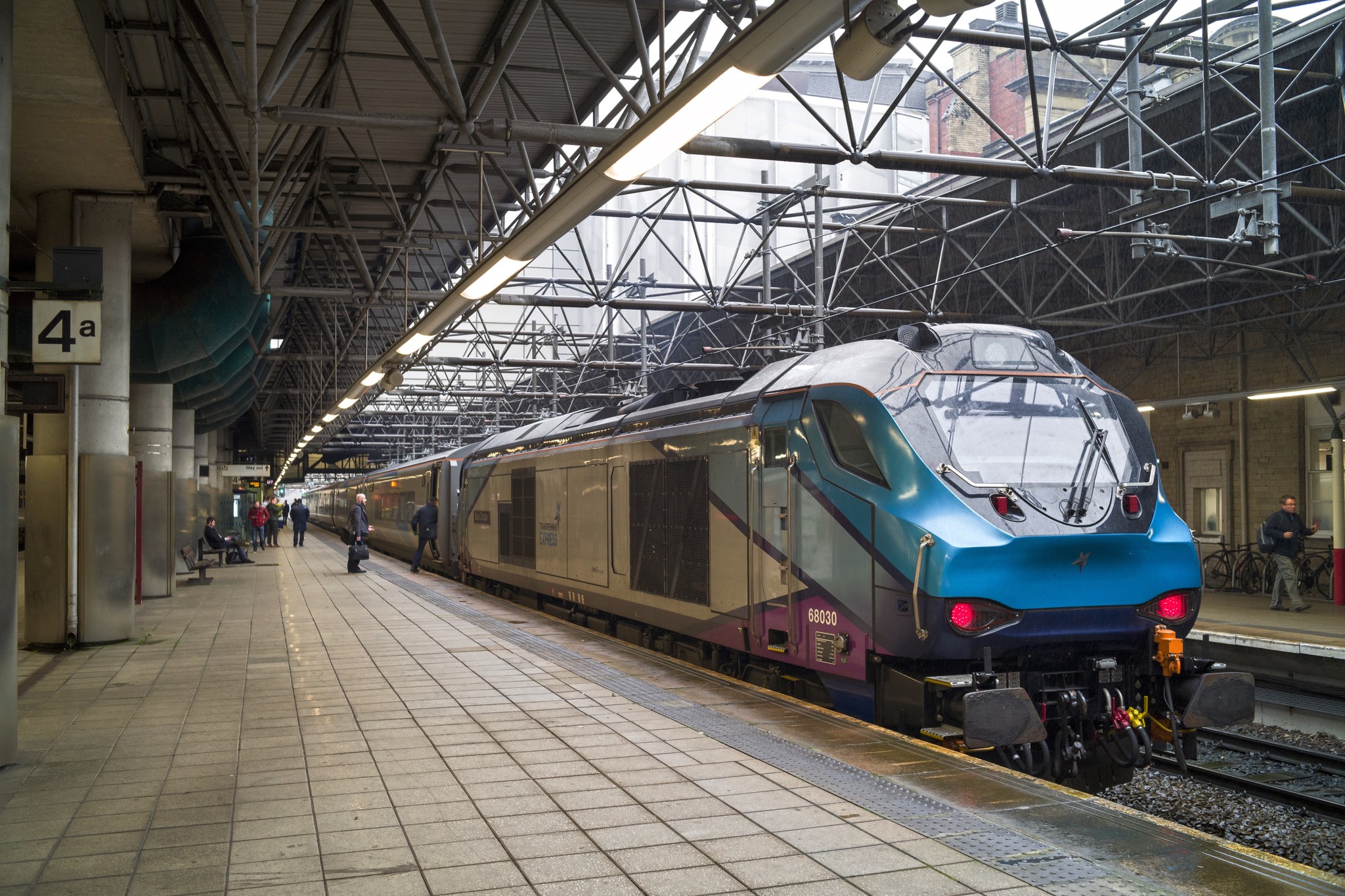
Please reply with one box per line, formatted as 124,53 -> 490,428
1201,536 -> 1333,597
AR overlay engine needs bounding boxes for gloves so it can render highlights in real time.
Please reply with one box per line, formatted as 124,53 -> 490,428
413,530 -> 419,535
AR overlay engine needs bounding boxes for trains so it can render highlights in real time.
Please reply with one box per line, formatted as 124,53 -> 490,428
295,322 -> 1256,794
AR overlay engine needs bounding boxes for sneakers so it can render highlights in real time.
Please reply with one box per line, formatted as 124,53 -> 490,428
261,544 -> 265,550
268,544 -> 273,547
274,544 -> 281,548
252,548 -> 258,553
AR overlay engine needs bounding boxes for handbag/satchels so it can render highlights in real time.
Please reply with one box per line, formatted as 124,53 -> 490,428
225,549 -> 248,565
1257,512 -> 1283,554
348,540 -> 369,560
340,528 -> 354,545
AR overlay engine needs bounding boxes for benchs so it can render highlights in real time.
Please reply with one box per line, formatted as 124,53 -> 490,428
198,536 -> 227,568
180,545 -> 214,586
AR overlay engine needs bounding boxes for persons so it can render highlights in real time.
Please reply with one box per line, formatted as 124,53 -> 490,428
346,494 -> 375,573
239,500 -> 248,537
247,499 -> 270,553
1262,494 -> 1319,613
289,499 -> 310,548
410,495 -> 438,572
204,516 -> 255,563
260,496 -> 298,547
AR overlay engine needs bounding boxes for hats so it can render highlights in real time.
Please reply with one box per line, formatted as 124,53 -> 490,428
296,498 -> 302,503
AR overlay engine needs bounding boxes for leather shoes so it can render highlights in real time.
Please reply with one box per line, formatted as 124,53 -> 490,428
234,541 -> 245,546
1295,604 -> 1312,611
243,559 -> 255,563
348,569 -> 354,572
410,566 -> 420,572
1270,607 -> 1290,611
353,566 -> 367,573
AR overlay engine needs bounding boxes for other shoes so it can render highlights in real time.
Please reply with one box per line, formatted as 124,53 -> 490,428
299,545 -> 304,547
293,545 -> 297,547
